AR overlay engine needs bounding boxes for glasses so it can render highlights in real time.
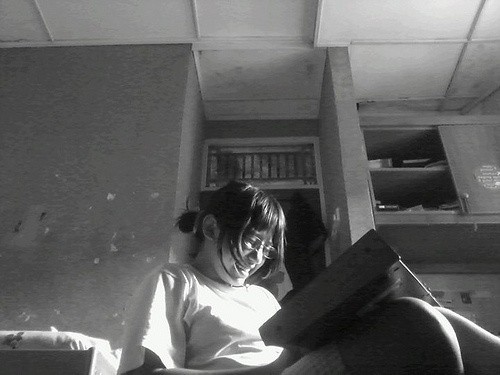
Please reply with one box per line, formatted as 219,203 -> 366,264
224,223 -> 277,261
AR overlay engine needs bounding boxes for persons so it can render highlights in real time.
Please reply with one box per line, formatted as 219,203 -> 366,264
116,177 -> 500,375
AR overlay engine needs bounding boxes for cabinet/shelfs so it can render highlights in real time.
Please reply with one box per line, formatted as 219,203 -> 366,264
357,111 -> 500,270
